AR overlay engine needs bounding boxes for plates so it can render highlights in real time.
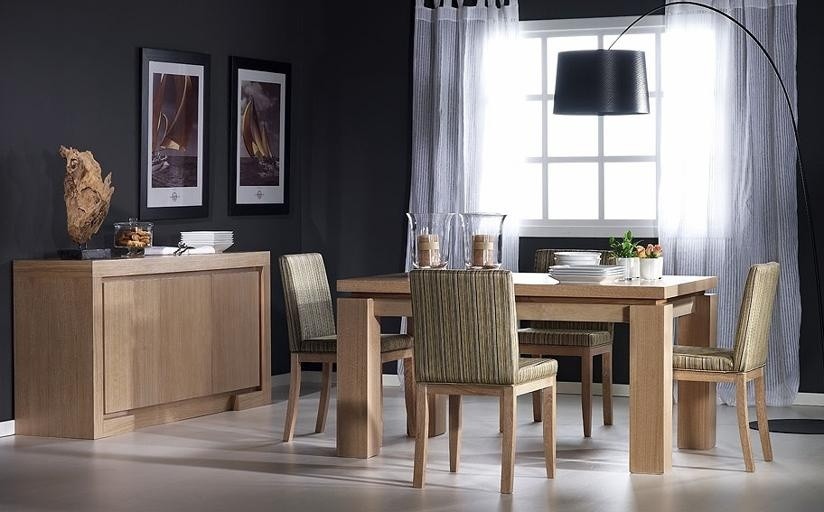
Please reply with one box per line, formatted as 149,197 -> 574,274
179,231 -> 234,253
548,264 -> 624,284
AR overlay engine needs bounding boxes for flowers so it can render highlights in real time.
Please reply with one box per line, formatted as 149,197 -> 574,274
638,244 -> 663,258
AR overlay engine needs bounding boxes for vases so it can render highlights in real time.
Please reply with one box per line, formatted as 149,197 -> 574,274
639,258 -> 662,280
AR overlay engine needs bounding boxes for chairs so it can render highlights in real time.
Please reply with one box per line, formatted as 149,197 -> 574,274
672,260 -> 780,473
408,269 -> 559,495
279,251 -> 416,443
518,247 -> 620,437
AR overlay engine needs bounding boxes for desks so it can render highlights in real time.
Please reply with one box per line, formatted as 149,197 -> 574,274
12,251 -> 272,439
336,273 -> 719,475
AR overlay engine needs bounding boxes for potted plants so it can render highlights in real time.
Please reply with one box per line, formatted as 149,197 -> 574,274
608,230 -> 640,279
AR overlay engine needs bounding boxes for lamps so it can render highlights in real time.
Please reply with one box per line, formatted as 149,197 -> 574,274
553,1 -> 823,435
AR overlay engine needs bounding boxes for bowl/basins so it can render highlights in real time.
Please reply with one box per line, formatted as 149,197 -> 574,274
554,252 -> 601,265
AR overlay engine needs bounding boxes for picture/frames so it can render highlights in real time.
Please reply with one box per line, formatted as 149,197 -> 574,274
140,46 -> 210,221
227,50 -> 293,217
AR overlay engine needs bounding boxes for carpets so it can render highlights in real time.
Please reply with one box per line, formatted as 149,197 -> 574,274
748,420 -> 824,434
